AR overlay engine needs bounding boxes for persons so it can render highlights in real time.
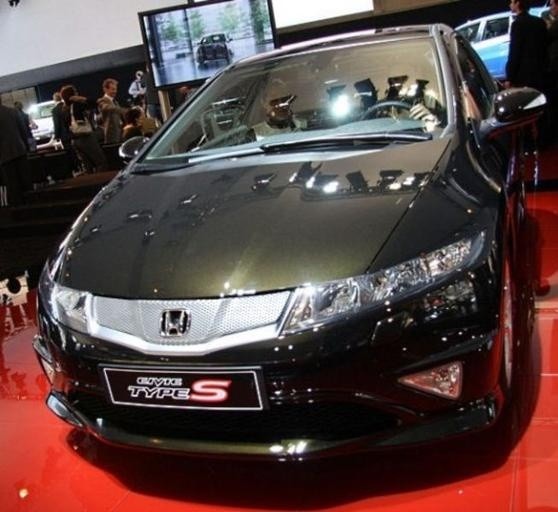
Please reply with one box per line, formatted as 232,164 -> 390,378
544,1 -> 557,148
13,100 -> 37,150
0,92 -> 33,201
368,55 -> 444,125
249,77 -> 314,142
501,0 -> 550,152
49,67 -> 191,176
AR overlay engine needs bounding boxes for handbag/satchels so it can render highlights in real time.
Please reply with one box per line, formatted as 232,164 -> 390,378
70,116 -> 94,135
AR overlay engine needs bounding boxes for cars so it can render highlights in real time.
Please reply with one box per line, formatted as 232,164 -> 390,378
32,24 -> 548,463
25,100 -> 64,151
195,32 -> 234,64
453,7 -> 552,81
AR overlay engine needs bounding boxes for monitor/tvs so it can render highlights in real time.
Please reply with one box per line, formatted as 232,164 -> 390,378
138,0 -> 280,91
